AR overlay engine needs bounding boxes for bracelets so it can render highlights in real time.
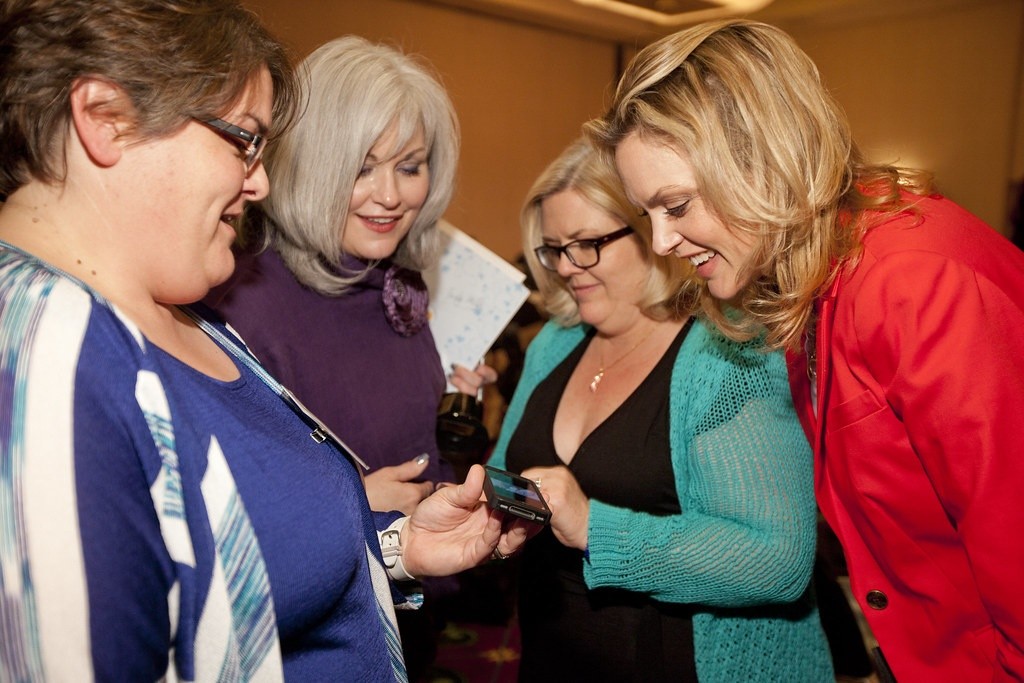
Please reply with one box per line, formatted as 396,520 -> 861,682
379,515 -> 415,581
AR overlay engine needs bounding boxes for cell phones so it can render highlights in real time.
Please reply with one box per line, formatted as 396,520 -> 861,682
483,464 -> 553,525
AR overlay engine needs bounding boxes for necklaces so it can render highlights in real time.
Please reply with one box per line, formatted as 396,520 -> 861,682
590,322 -> 660,393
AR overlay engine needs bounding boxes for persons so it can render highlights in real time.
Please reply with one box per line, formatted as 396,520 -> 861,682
187,35 -> 497,683
0,0 -> 553,683
583,18 -> 1024,683
436,135 -> 838,683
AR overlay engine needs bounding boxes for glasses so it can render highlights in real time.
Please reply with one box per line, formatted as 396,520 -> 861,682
191,110 -> 268,171
534,226 -> 636,272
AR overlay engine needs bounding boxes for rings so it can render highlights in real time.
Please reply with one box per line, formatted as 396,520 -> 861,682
492,547 -> 509,560
534,478 -> 541,488
